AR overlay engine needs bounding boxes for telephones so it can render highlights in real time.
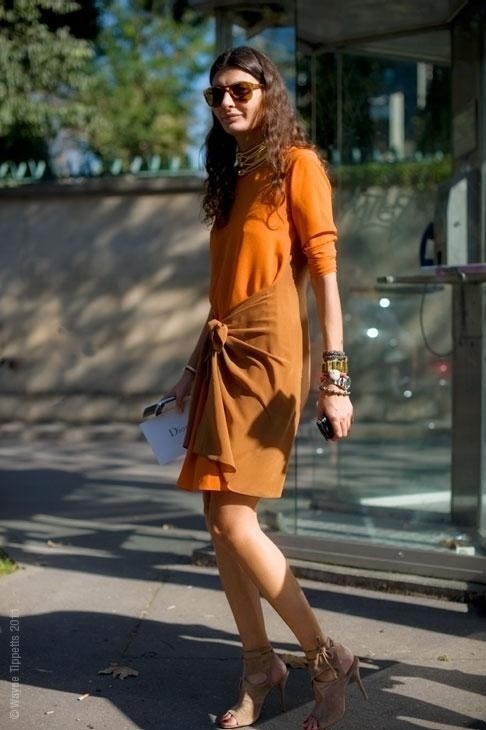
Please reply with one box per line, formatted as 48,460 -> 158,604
419,170 -> 477,268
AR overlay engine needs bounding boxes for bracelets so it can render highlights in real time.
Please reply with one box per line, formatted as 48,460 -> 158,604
185,365 -> 197,376
317,350 -> 351,396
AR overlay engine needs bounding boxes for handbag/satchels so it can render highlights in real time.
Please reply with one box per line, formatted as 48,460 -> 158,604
138,401 -> 195,467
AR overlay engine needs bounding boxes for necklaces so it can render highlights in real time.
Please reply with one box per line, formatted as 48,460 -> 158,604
236,143 -> 268,177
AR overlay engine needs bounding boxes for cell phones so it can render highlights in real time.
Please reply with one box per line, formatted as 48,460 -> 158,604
155,393 -> 191,416
317,415 -> 335,442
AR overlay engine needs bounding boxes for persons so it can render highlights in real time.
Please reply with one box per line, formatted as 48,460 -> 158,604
160,46 -> 369,730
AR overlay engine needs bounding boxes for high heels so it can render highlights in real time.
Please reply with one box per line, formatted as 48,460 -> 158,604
216,646 -> 291,728
298,638 -> 370,728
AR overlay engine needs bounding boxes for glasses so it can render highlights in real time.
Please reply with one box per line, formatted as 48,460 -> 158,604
203,82 -> 264,109
